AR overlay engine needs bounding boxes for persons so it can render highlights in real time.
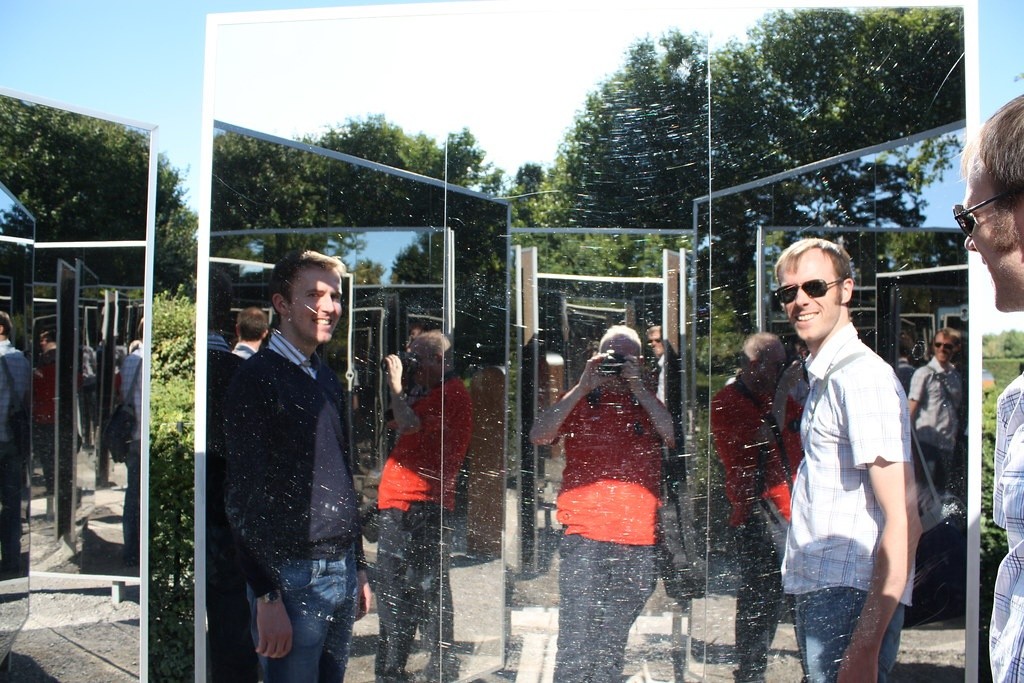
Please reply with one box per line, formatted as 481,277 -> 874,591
210,267 -> 504,683
897,328 -> 964,503
954,93 -> 1024,683
82,332 -> 139,449
223,250 -> 373,683
29,326 -> 57,522
0,311 -> 31,581
114,317 -> 146,566
545,324 -> 697,681
713,235 -> 924,683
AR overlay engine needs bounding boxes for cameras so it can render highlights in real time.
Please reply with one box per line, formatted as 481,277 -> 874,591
600,354 -> 626,376
380,351 -> 421,379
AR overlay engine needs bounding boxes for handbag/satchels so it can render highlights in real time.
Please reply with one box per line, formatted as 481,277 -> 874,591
105,402 -> 136,461
658,503 -> 707,598
406,501 -> 451,573
751,499 -> 788,575
9,394 -> 29,461
33,397 -> 55,425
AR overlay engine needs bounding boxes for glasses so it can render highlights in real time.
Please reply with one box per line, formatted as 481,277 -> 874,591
953,187 -> 1023,237
935,342 -> 954,350
775,279 -> 841,304
